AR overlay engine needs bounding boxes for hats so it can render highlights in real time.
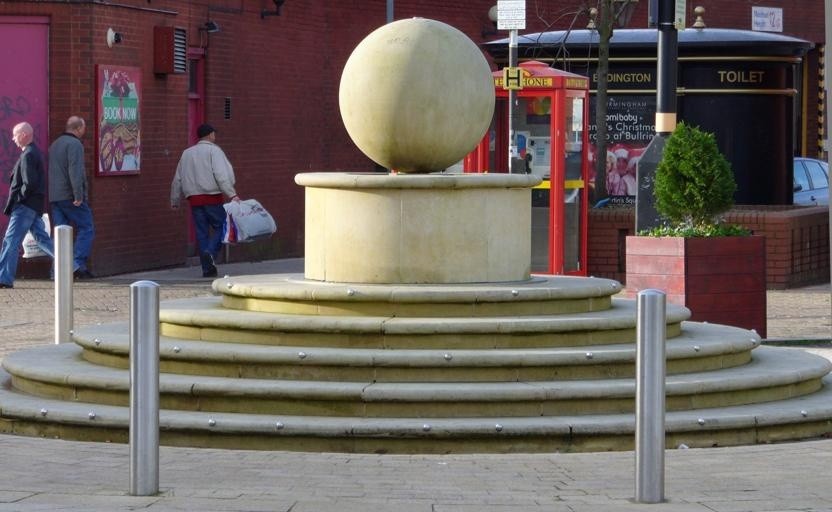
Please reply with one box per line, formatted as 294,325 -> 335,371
792,157 -> 830,206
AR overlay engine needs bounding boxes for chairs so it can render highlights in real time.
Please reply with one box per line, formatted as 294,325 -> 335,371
201,251 -> 218,277
0,270 -> 95,288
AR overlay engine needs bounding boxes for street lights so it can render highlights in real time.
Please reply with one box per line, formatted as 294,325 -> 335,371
220,198 -> 277,248
22,213 -> 52,259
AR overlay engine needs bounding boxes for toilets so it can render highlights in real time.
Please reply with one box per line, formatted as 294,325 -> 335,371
526,136 -> 551,178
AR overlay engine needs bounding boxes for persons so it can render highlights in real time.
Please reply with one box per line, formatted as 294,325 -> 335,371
616,147 -> 627,176
606,151 -> 622,196
170,124 -> 241,277
0,121 -> 84,290
48,115 -> 96,278
618,156 -> 638,196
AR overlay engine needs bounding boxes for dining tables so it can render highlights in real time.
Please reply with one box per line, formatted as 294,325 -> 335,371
624,120 -> 767,338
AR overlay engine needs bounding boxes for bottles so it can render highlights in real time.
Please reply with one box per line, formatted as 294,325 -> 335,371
197,124 -> 218,138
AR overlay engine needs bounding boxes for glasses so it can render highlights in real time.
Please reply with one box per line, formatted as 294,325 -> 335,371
199,22 -> 220,34
